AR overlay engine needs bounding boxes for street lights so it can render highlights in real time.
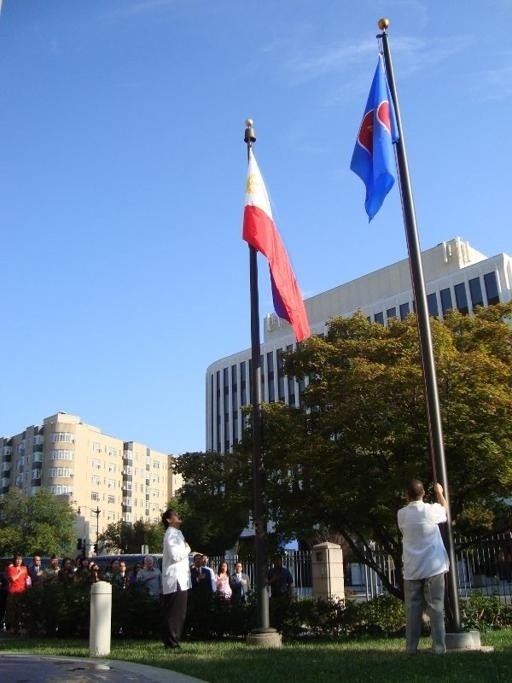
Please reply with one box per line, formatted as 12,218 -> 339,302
76,505 -> 102,556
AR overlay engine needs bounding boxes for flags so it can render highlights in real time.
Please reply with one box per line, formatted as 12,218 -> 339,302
242,147 -> 310,343
349,53 -> 400,223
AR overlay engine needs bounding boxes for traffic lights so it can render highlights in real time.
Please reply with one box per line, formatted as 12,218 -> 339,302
77,538 -> 82,550
93,544 -> 98,553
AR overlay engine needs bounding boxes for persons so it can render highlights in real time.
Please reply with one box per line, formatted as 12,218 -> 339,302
0,509 -> 251,648
269,554 -> 293,598
397,478 -> 449,656
